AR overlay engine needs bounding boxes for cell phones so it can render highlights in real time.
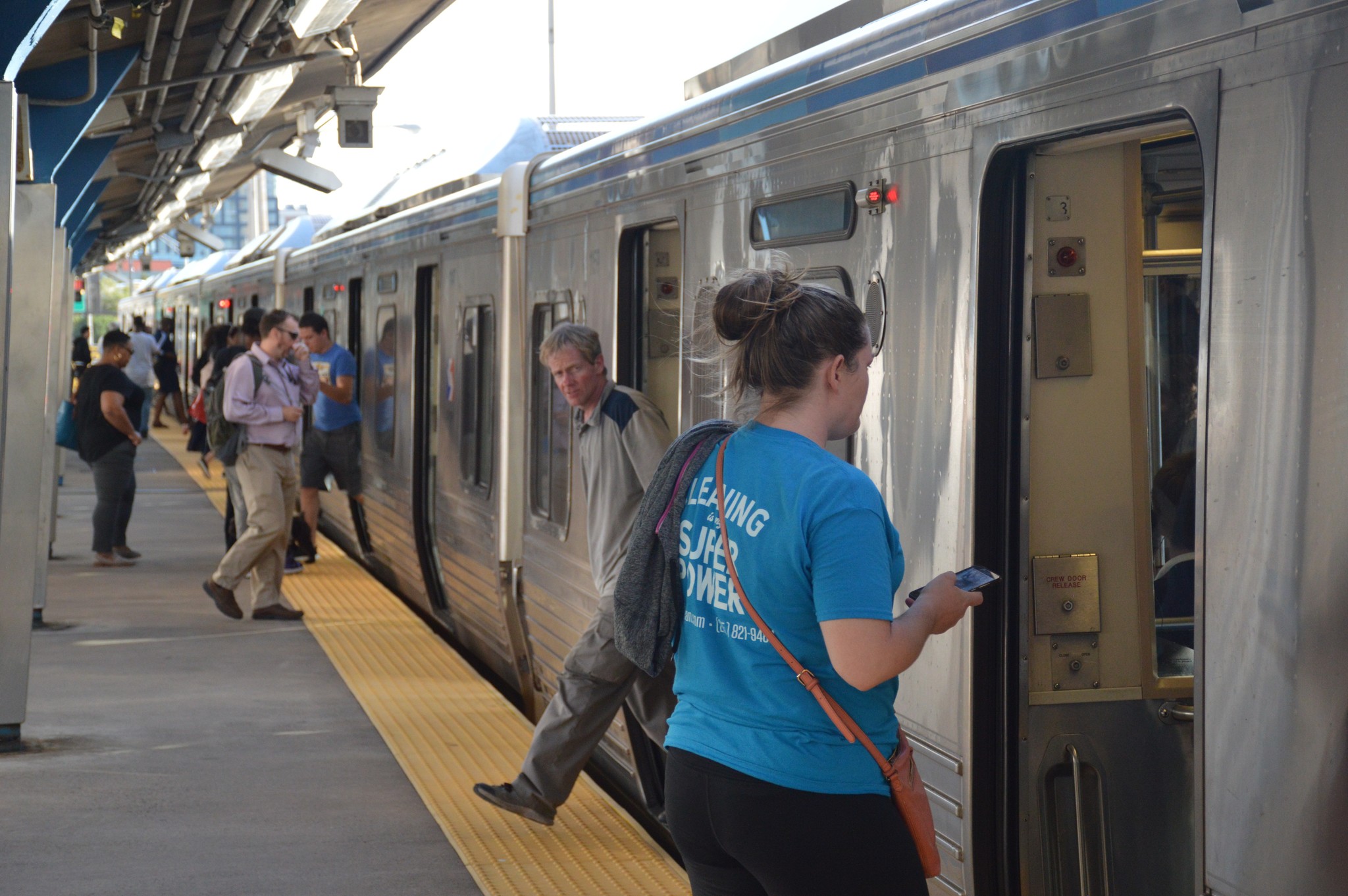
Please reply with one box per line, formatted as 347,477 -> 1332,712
909,566 -> 1003,604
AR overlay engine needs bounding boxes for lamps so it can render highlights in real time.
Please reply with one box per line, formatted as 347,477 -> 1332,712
276,0 -> 362,38
102,0 -> 137,41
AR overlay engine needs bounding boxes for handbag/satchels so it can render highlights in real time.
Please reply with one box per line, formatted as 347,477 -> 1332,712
188,392 -> 207,425
205,352 -> 262,466
54,400 -> 79,452
872,730 -> 941,878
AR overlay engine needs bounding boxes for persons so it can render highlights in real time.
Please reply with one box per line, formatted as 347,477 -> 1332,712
192,308 -> 361,622
74,327 -> 145,568
661,266 -> 984,896
1151,449 -> 1196,677
362,317 -> 399,450
72,316 -> 188,439
473,325 -> 671,826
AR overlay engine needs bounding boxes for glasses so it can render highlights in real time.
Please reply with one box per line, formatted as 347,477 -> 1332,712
123,346 -> 135,356
227,321 -> 233,337
275,325 -> 298,341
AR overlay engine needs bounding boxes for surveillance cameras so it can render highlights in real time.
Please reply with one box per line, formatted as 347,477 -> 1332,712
176,220 -> 228,252
249,148 -> 344,196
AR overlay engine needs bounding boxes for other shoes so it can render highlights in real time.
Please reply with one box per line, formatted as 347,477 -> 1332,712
114,546 -> 140,561
252,603 -> 302,622
204,578 -> 242,620
284,560 -> 301,572
474,781 -> 554,828
94,552 -> 136,566
